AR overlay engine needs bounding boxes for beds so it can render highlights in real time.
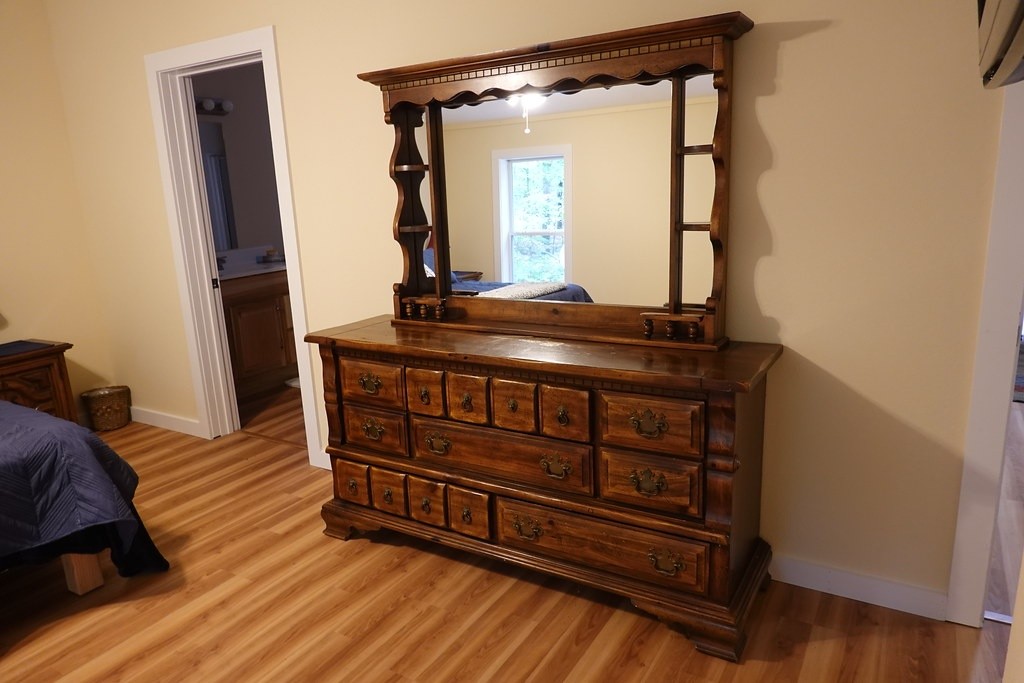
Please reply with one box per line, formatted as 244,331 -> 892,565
422,231 -> 596,303
0,401 -> 170,596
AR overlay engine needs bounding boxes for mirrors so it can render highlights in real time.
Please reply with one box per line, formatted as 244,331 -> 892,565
197,121 -> 238,252
355,11 -> 754,352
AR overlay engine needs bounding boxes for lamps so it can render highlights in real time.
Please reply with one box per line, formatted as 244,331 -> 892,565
194,98 -> 234,117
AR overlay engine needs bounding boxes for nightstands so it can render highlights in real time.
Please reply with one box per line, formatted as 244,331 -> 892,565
451,271 -> 484,281
0,337 -> 80,427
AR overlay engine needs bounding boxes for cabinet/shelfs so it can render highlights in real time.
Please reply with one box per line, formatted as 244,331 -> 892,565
303,314 -> 784,664
219,269 -> 298,398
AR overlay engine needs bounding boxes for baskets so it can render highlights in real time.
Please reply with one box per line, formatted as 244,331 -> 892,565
80,386 -> 130,431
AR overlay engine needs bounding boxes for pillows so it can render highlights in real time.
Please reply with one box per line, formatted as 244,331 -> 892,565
423,249 -> 457,283
